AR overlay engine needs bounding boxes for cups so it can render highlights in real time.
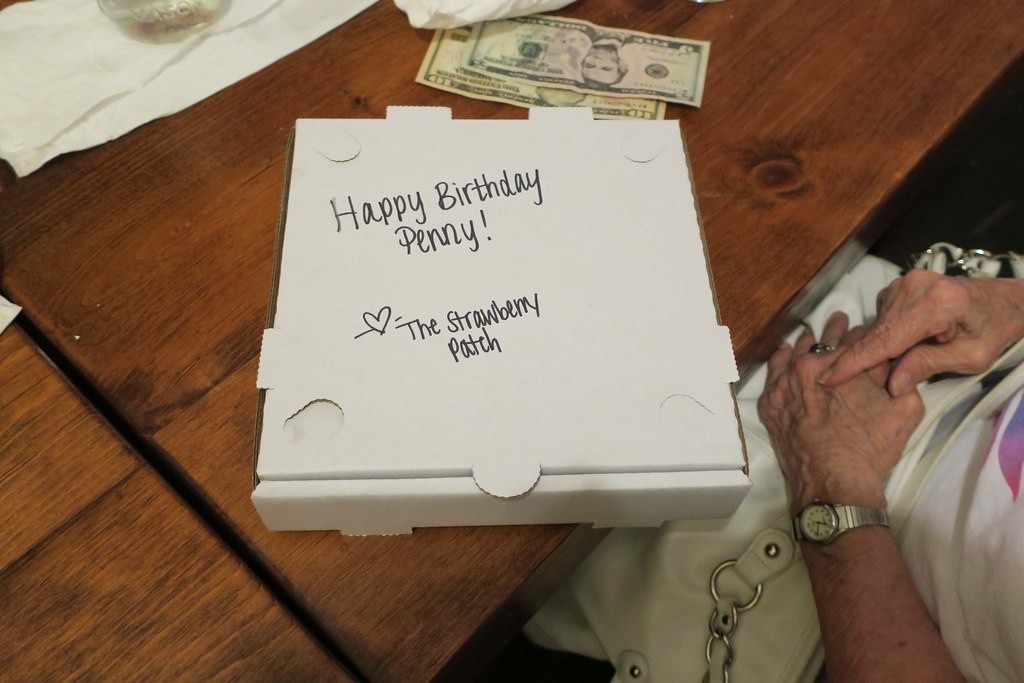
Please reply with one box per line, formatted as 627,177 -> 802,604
98,0 -> 225,44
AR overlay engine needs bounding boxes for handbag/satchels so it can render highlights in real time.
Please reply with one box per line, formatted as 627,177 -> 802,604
519,253 -> 979,683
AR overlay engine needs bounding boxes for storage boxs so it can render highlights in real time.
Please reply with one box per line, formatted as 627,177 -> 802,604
251,106 -> 753,528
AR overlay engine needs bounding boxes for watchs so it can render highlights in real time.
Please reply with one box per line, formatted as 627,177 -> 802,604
790,498 -> 890,546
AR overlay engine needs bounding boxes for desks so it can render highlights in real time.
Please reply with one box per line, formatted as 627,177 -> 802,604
0,0 -> 1024,683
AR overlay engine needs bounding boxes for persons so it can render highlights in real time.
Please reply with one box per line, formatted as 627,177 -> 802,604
462,241 -> 1024,683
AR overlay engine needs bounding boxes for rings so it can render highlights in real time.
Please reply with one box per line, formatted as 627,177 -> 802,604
808,344 -> 835,353
